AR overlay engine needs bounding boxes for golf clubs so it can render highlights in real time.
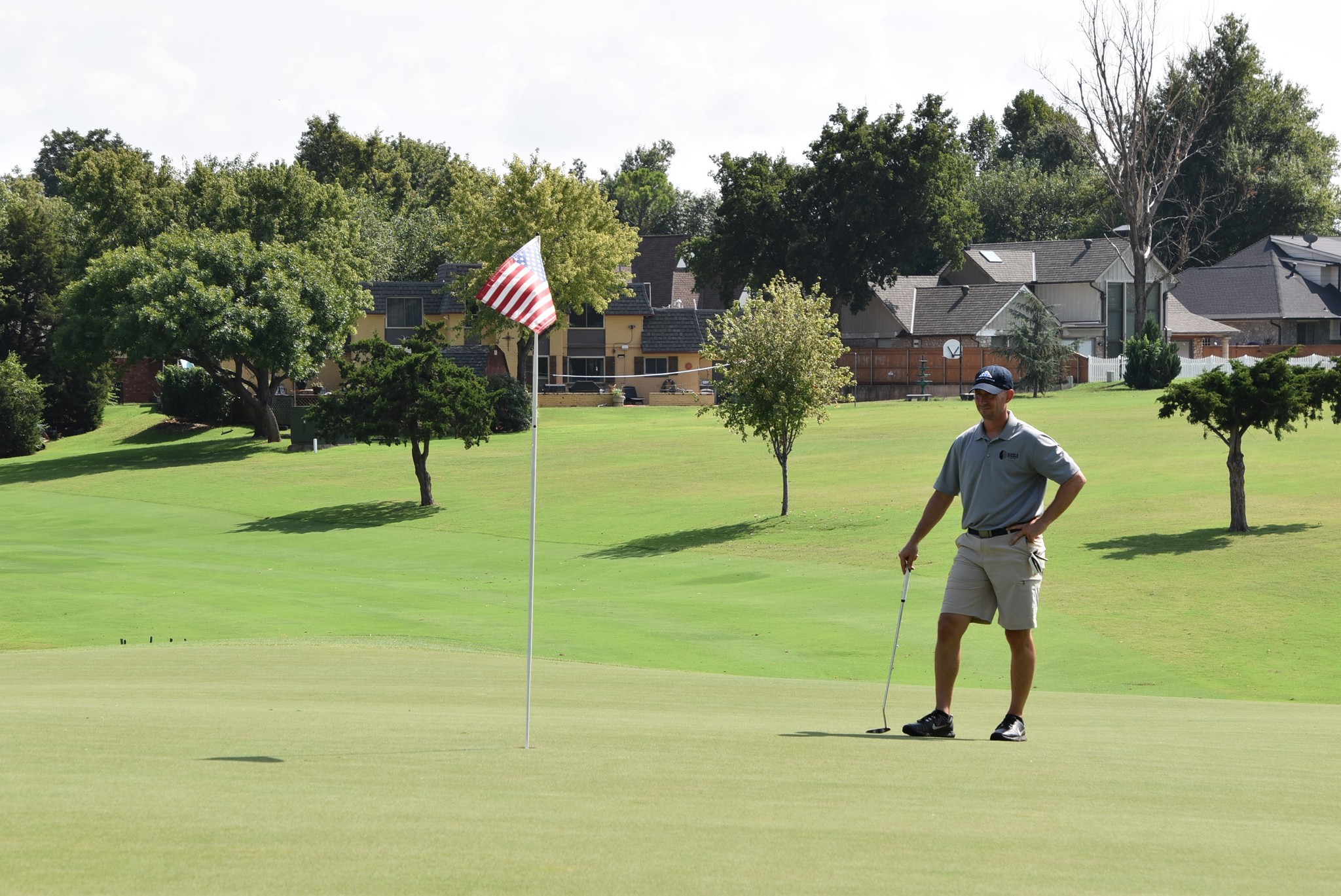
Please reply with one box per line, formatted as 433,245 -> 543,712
865,566 -> 912,734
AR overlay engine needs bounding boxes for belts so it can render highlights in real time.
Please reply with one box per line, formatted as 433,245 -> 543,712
967,526 -> 1021,538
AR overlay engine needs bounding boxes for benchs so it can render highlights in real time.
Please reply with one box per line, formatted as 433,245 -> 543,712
959,393 -> 975,401
906,394 -> 932,401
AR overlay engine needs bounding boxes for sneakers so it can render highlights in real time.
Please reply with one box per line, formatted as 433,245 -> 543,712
990,714 -> 1027,742
902,710 -> 955,738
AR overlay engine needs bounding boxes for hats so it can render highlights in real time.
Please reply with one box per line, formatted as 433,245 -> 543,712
969,364 -> 1013,395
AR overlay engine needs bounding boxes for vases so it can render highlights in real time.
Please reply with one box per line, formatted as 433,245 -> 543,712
296,379 -> 307,390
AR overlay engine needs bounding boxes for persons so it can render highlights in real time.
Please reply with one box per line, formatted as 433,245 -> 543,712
898,365 -> 1086,741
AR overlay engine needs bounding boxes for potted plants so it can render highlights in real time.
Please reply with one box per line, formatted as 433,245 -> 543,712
611,385 -> 625,403
311,380 -> 324,395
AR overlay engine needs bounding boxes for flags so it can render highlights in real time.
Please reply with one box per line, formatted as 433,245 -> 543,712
474,232 -> 557,335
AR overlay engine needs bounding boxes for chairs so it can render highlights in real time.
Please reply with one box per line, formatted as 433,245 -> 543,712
623,386 -> 645,405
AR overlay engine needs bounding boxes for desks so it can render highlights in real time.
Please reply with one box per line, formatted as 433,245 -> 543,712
659,389 -> 685,393
